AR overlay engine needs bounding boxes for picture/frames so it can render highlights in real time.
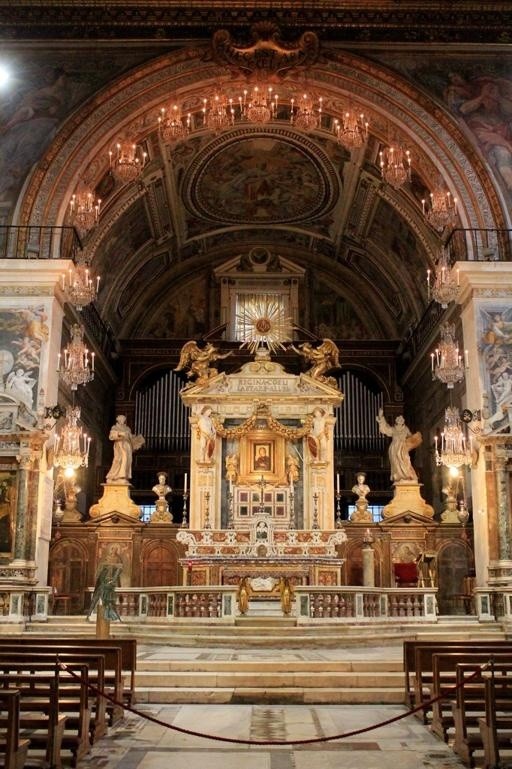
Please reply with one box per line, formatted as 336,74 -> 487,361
238,428 -> 287,486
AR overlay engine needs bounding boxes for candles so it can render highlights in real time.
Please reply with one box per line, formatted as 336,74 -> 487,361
336,473 -> 341,497
183,473 -> 189,494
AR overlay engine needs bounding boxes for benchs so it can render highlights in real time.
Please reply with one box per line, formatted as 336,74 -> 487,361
402,637 -> 512,768
0,631 -> 137,769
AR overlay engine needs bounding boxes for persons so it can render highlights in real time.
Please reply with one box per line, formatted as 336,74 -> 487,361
11,336 -> 40,362
286,451 -> 300,481
206,147 -> 315,219
42,421 -> 58,459
5,368 -> 37,402
430,52 -> 512,194
486,344 -> 505,369
225,452 -> 237,481
0,63 -> 93,148
488,358 -> 511,383
106,415 -> 145,483
351,472 -> 370,499
197,406 -> 216,462
305,405 -> 333,464
152,471 -> 172,499
280,577 -> 298,619
236,577 -> 251,617
395,544 -> 419,565
254,447 -> 269,470
173,340 -> 233,381
480,312 -> 506,346
103,545 -> 125,569
486,371 -> 512,403
0,477 -> 14,520
288,337 -> 341,387
377,407 -> 422,481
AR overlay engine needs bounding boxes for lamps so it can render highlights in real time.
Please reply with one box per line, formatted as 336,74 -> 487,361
108,140 -> 148,181
379,145 -> 413,190
334,109 -> 370,151
421,191 -> 459,232
290,93 -> 323,134
157,102 -> 193,145
67,181 -> 102,238
238,84 -> 279,127
51,261 -> 101,479
424,261 -> 472,480
202,91 -> 236,135
